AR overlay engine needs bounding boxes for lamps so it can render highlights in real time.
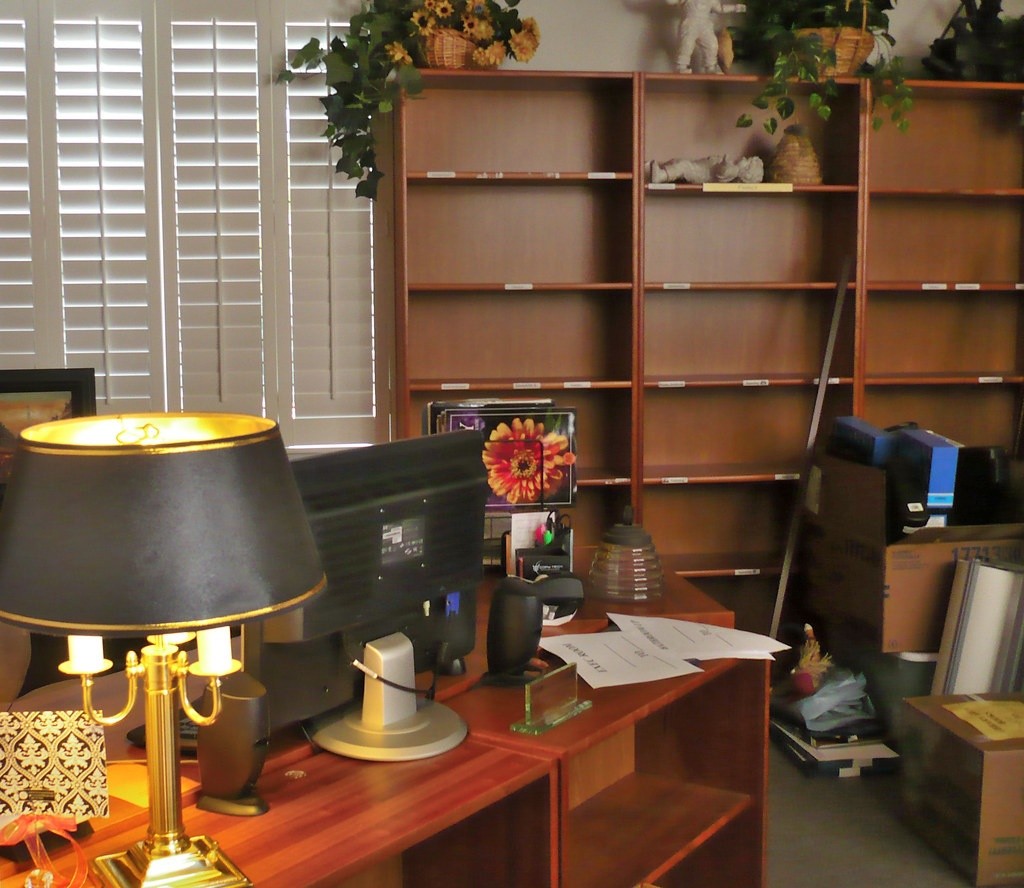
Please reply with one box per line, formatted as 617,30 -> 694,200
0,414 -> 331,888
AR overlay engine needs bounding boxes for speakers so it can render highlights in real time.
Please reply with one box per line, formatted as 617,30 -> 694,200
193,671 -> 274,816
480,577 -> 546,689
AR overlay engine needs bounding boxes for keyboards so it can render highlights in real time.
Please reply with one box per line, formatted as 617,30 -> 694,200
124,693 -> 206,758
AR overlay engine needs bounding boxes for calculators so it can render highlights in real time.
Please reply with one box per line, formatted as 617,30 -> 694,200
542,601 -> 578,627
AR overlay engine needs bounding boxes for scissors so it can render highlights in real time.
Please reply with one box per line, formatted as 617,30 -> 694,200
546,508 -> 573,554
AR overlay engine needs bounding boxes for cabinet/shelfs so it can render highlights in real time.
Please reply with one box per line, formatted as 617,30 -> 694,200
393,68 -> 1024,671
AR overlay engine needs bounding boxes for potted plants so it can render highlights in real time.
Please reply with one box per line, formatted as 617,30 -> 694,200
275,1 -> 539,204
717,0 -> 916,139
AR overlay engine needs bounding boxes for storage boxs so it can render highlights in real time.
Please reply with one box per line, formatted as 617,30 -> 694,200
897,692 -> 1024,888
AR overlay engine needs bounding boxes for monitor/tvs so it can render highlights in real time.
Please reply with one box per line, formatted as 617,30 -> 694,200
236,428 -> 485,763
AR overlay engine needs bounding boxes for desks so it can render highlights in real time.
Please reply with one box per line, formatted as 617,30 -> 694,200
0,604 -> 773,888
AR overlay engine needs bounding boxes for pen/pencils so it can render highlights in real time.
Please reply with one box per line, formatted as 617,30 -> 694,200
536,523 -> 546,556
543,530 -> 553,556
